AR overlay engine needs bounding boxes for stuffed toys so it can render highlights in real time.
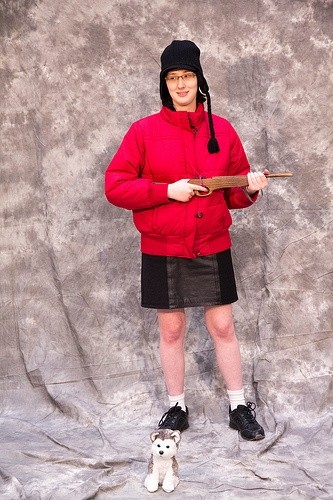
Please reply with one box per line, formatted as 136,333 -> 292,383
145,428 -> 181,493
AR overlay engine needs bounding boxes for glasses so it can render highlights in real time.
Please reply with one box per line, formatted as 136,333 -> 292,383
164,73 -> 196,81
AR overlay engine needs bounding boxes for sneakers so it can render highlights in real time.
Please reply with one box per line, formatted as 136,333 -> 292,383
157,402 -> 189,434
228,402 -> 265,441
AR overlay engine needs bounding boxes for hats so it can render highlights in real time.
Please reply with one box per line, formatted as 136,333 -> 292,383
159,40 -> 220,153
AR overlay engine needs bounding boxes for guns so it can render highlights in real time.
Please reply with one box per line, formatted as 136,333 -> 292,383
152,173 -> 293,198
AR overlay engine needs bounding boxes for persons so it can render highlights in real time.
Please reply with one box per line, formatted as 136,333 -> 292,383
104,40 -> 270,441
